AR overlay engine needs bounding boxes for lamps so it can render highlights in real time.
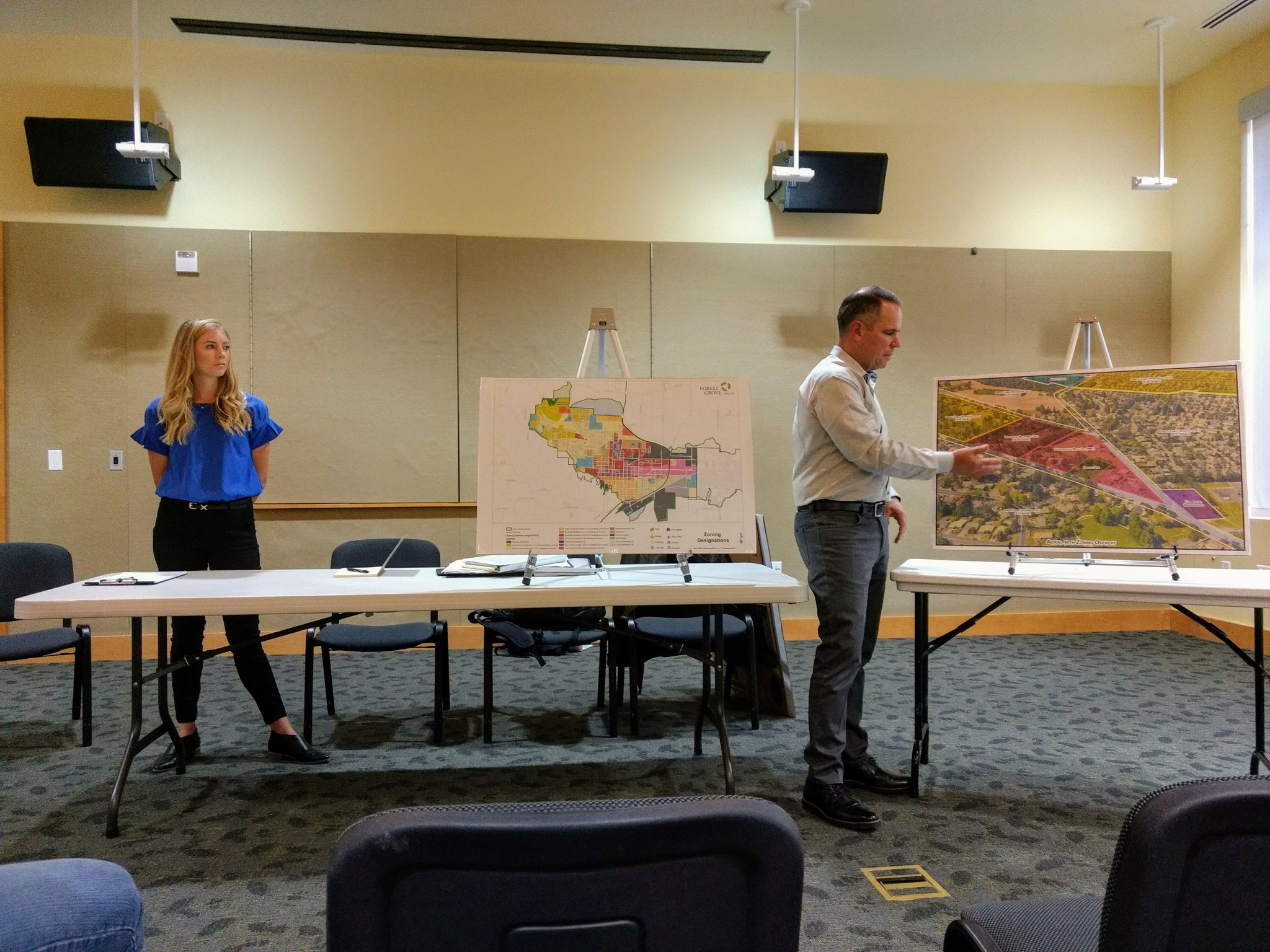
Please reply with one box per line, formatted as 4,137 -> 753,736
1131,14 -> 1179,191
771,3 -> 816,182
117,2 -> 168,159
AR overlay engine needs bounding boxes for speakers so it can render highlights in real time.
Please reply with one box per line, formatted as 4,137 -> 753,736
770,150 -> 889,214
24,116 -> 175,190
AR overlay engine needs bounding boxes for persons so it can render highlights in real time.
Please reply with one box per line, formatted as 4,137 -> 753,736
796,286 -> 1004,830
130,318 -> 332,772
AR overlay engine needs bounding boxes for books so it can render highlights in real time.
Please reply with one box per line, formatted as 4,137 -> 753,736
441,554 -> 596,576
332,534 -> 407,577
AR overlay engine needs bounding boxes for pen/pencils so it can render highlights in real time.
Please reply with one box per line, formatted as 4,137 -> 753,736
346,568 -> 370,573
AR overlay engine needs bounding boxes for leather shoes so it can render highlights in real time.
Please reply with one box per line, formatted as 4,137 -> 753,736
801,775 -> 881,829
152,727 -> 201,772
267,730 -> 330,763
842,756 -> 911,793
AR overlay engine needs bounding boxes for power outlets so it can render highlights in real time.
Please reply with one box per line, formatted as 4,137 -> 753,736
109,449 -> 123,471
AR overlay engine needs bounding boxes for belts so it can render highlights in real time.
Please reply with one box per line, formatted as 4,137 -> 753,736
181,497 -> 252,510
797,500 -> 887,517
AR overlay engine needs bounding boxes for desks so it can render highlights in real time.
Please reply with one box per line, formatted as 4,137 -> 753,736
13,563 -> 805,839
889,558 -> 1270,799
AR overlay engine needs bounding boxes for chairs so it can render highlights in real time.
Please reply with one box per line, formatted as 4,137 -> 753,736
303,540 -> 452,749
481,552 -> 606,742
944,772 -> 1269,952
0,541 -> 99,744
611,550 -> 764,732
326,796 -> 813,952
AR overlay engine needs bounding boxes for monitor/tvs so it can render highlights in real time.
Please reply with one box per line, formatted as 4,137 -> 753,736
930,361 -> 1250,555
